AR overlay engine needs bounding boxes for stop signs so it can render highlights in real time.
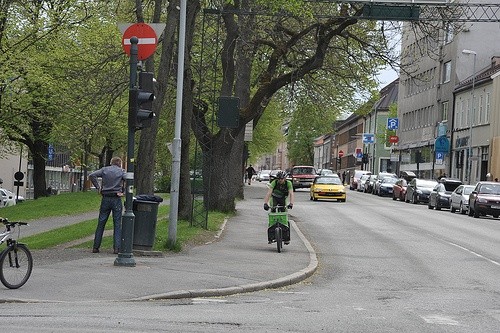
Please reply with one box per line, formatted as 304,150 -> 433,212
339,151 -> 343,156
122,23 -> 158,60
390,135 -> 398,143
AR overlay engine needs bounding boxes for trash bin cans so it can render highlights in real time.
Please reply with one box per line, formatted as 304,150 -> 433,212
131,199 -> 160,250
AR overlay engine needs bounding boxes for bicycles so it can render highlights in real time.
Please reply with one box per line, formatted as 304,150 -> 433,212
0,217 -> 33,289
264,203 -> 291,253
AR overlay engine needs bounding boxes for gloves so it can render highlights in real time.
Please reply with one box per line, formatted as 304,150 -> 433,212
264,204 -> 269,211
287,203 -> 293,210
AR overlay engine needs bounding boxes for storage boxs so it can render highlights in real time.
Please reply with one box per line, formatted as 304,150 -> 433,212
268,212 -> 289,227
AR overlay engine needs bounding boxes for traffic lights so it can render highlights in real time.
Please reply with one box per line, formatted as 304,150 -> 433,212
129,89 -> 156,131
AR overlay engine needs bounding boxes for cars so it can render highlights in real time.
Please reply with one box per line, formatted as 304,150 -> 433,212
350,170 -> 408,202
429,178 -> 464,210
252,170 -> 292,183
310,175 -> 347,202
404,177 -> 439,204
449,184 -> 477,214
0,188 -> 25,206
468,181 -> 500,218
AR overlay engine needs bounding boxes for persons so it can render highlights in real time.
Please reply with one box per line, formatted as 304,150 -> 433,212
263,171 -> 294,244
89,157 -> 128,254
437,172 -> 446,182
246,164 -> 256,185
342,170 -> 346,185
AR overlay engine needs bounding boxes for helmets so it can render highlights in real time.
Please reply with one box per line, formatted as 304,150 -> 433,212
276,171 -> 287,179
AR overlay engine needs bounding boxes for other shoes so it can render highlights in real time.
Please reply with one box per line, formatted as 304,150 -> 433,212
92,248 -> 99,253
113,248 -> 119,254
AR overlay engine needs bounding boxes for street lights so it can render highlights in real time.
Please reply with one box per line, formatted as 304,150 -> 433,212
462,49 -> 477,184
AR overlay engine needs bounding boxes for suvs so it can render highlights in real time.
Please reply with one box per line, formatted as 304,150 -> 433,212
287,165 -> 319,191
